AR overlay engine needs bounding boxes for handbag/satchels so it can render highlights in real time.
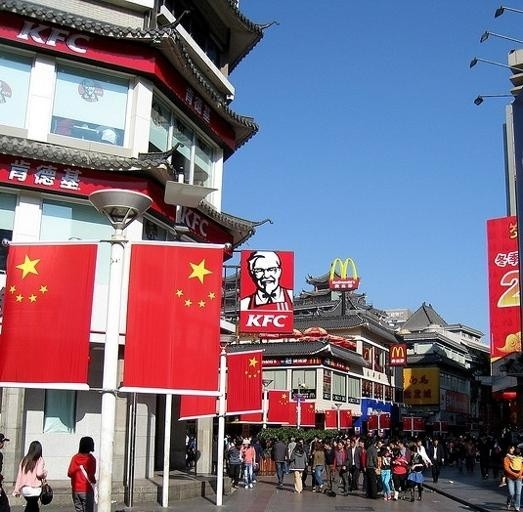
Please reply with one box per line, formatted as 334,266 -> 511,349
39,478 -> 53,506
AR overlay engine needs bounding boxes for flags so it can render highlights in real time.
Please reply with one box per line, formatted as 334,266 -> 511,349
268,391 -> 289,422
340,411 -> 353,428
402,418 -> 425,430
289,403 -> 296,425
1,244 -> 98,383
240,413 -> 264,421
227,351 -> 263,412
118,240 -> 223,391
326,410 -> 338,426
300,403 -> 316,426
179,396 -> 216,417
368,415 -> 391,429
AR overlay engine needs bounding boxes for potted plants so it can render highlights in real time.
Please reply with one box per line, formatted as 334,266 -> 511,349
256,427 -> 285,478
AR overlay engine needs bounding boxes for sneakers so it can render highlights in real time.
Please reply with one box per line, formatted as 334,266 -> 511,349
365,490 -> 423,503
243,478 -> 359,498
480,473 -> 521,512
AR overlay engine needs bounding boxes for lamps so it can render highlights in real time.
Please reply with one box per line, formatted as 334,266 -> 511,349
493,4 -> 523,21
468,56 -> 522,73
471,91 -> 521,106
478,29 -> 522,48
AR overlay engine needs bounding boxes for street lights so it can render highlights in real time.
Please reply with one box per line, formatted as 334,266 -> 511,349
86,187 -> 155,512
214,333 -> 239,505
261,375 -> 477,439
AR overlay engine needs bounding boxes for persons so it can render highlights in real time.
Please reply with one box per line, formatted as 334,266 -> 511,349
68,437 -> 96,511
79,79 -> 103,104
240,251 -> 293,312
55,118 -> 74,136
96,125 -> 120,146
0,81 -> 12,104
12,441 -> 47,512
0,433 -> 11,512
183,432 -> 196,468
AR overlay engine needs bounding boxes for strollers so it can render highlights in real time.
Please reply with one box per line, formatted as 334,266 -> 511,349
399,463 -> 426,502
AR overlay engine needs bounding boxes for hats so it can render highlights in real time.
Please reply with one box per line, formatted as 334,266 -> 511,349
0,433 -> 10,442
242,438 -> 251,445
